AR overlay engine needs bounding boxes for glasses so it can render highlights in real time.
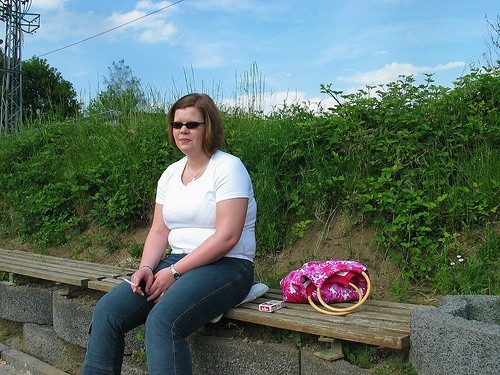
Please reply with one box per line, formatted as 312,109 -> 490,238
170,121 -> 207,129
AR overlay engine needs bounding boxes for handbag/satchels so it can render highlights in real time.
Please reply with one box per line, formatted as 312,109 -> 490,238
280,260 -> 371,315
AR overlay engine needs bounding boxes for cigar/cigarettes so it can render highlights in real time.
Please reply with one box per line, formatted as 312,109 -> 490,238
123,278 -> 141,289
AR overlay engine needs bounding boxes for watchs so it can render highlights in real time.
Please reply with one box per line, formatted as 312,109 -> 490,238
171,264 -> 180,280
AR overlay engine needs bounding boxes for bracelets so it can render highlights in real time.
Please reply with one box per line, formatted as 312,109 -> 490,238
139,265 -> 153,271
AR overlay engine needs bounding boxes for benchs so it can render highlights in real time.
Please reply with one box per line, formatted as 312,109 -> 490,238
88,268 -> 436,351
0,248 -> 138,293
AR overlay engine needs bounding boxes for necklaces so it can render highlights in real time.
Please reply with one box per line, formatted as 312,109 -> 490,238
187,159 -> 208,180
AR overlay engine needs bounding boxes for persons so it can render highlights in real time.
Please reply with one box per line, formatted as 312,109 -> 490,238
82,93 -> 258,374
